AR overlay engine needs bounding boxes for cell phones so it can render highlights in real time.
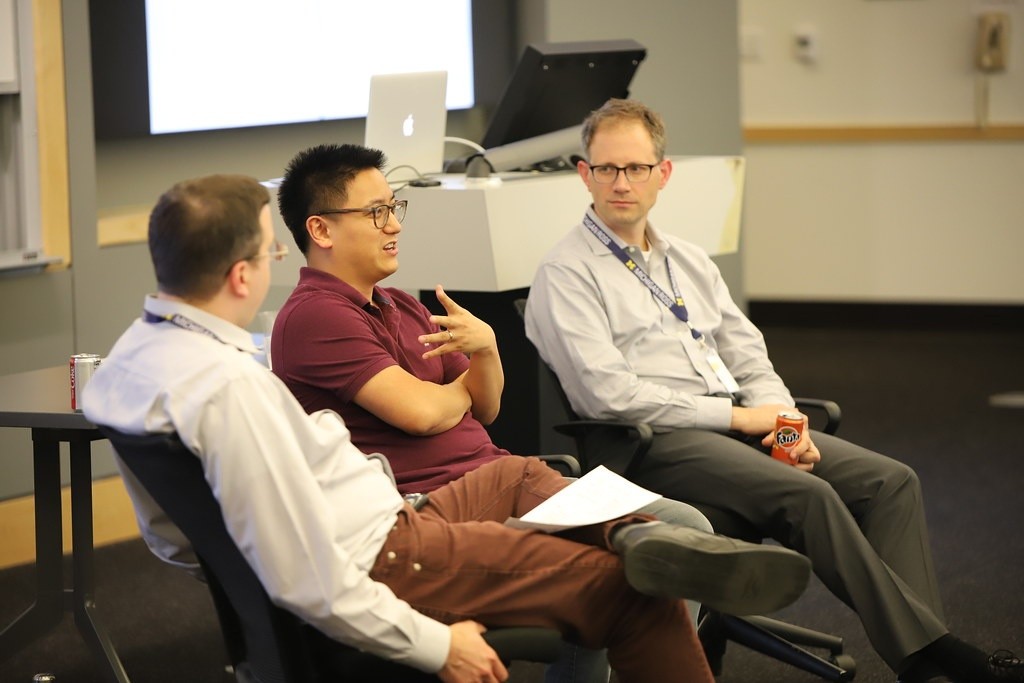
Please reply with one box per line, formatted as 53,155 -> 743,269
409,180 -> 441,187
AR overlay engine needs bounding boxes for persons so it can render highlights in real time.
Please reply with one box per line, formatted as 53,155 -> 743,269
80,178 -> 811,682
525,98 -> 1024,683
271,144 -> 715,683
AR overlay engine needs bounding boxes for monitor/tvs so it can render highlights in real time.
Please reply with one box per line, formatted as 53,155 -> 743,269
483,40 -> 647,150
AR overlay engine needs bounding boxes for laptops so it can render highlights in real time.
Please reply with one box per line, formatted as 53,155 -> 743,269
364,69 -> 448,183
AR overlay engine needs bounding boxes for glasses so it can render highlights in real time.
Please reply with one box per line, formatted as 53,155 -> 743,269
224,239 -> 287,277
316,200 -> 409,229
588,161 -> 660,185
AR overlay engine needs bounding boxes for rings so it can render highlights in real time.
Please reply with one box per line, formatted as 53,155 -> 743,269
446,329 -> 453,342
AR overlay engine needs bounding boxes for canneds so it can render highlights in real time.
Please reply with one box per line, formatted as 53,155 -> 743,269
771,411 -> 804,465
69,353 -> 102,411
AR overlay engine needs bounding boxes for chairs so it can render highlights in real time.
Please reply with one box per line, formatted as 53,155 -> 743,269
2,299 -> 855,683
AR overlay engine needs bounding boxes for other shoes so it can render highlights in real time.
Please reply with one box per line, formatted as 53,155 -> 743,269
622,522 -> 811,615
988,648 -> 1024,683
